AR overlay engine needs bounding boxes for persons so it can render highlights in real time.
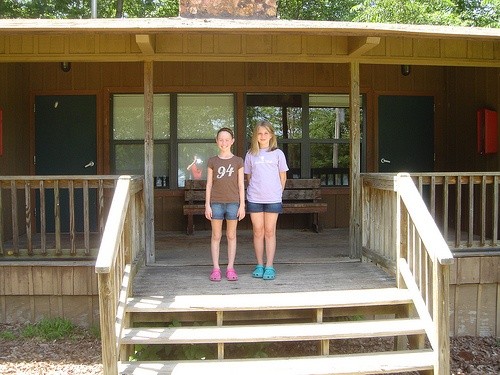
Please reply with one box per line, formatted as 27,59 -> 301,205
204,127 -> 245,282
186,153 -> 204,181
243,120 -> 289,280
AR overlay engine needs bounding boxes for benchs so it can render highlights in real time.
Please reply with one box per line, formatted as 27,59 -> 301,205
182,179 -> 329,235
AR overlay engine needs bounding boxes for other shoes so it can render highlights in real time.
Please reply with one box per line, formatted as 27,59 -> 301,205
252,265 -> 275,280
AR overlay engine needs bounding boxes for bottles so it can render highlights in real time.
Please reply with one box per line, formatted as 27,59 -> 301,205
162,174 -> 167,187
156,176 -> 162,187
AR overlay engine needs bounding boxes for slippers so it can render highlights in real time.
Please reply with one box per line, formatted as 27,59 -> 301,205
209,268 -> 222,281
225,268 -> 239,280
